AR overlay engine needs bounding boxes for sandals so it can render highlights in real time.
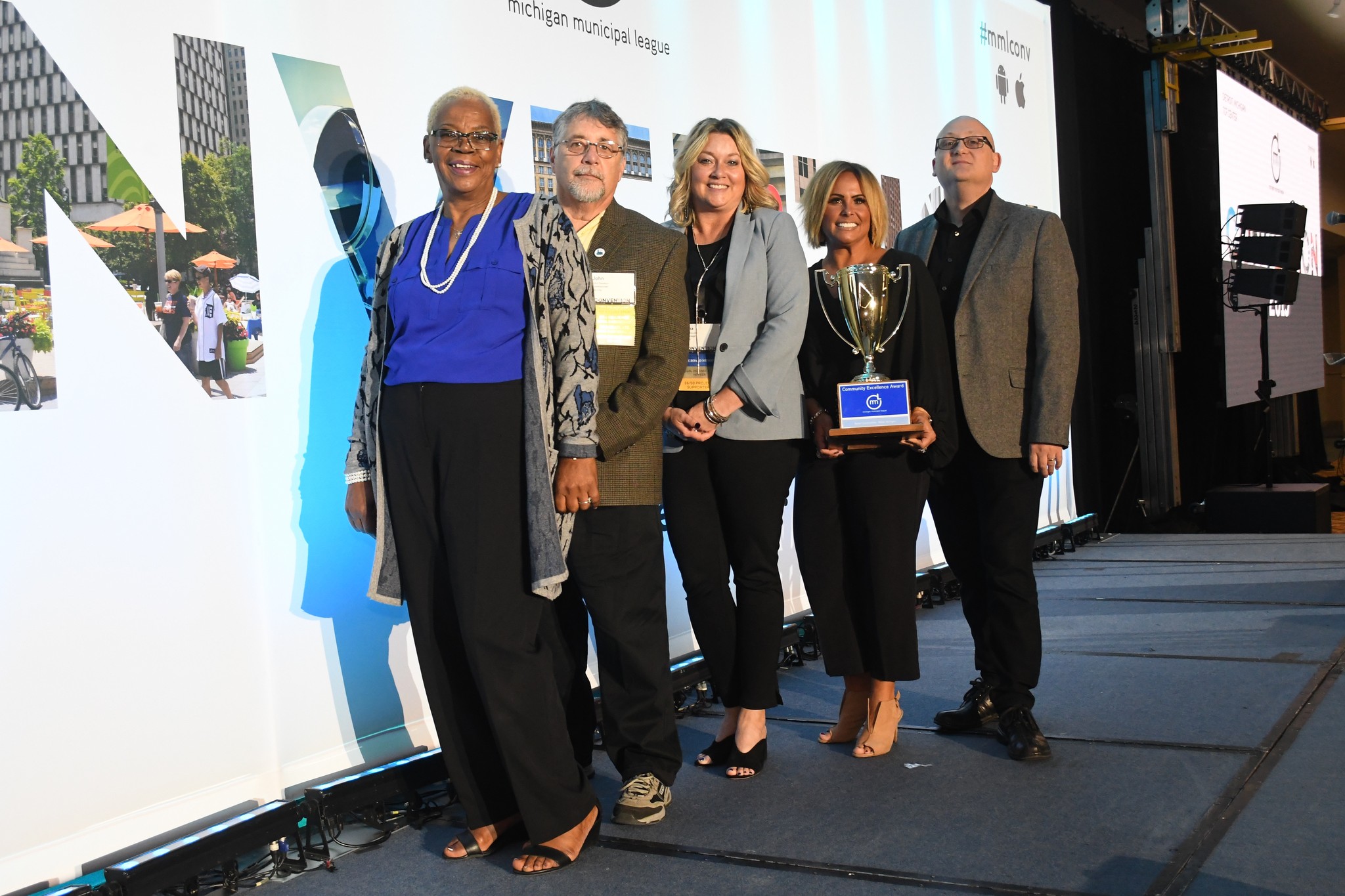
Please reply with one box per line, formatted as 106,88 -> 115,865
442,811 -> 529,861
512,797 -> 604,877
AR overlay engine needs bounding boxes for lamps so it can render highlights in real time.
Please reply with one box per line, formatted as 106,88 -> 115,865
48,748 -> 462,896
592,511 -> 1104,750
1226,201 -> 1307,488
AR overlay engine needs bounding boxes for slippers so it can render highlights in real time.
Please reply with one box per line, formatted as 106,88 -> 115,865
694,730 -> 735,768
725,732 -> 767,780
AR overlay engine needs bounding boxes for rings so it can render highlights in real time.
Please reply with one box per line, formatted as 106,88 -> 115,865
1048,458 -> 1055,461
578,497 -> 592,504
915,447 -> 927,453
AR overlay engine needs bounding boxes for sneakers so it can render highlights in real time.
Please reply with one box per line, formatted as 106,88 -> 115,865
610,771 -> 673,826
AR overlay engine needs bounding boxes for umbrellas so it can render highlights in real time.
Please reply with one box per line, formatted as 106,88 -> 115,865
0,203 -> 237,296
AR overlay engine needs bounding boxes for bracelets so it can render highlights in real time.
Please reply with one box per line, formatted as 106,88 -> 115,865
809,408 -> 829,424
344,469 -> 372,484
911,410 -> 933,425
703,393 -> 731,427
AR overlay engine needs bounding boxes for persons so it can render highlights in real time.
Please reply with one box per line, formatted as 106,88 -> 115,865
343,86 -> 602,875
796,160 -> 959,756
244,292 -> 261,314
660,116 -> 811,780
223,296 -> 240,313
549,100 -> 690,827
186,264 -> 238,399
892,114 -> 1081,762
156,269 -> 195,381
226,287 -> 236,304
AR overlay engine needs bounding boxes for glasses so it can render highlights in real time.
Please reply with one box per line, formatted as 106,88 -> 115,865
554,138 -> 624,157
935,136 -> 995,153
430,128 -> 498,151
195,276 -> 206,281
164,279 -> 178,283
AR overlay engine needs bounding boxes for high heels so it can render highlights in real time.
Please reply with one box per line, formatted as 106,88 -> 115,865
851,690 -> 904,757
818,687 -> 870,744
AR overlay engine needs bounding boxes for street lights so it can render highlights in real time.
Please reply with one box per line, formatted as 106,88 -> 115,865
62,186 -> 70,220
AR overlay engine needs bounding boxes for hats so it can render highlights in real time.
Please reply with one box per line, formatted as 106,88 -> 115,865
193,265 -> 211,276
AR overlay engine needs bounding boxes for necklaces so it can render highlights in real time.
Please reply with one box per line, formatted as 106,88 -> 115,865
692,220 -> 727,271
420,186 -> 498,294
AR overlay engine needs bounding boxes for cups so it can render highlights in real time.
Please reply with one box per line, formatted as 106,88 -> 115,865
188,295 -> 197,310
154,302 -> 163,318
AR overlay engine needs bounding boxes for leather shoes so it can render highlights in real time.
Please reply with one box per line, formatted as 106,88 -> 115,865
993,704 -> 1055,761
932,675 -> 1001,730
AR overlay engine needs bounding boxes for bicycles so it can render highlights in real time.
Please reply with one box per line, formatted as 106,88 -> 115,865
0,312 -> 41,411
